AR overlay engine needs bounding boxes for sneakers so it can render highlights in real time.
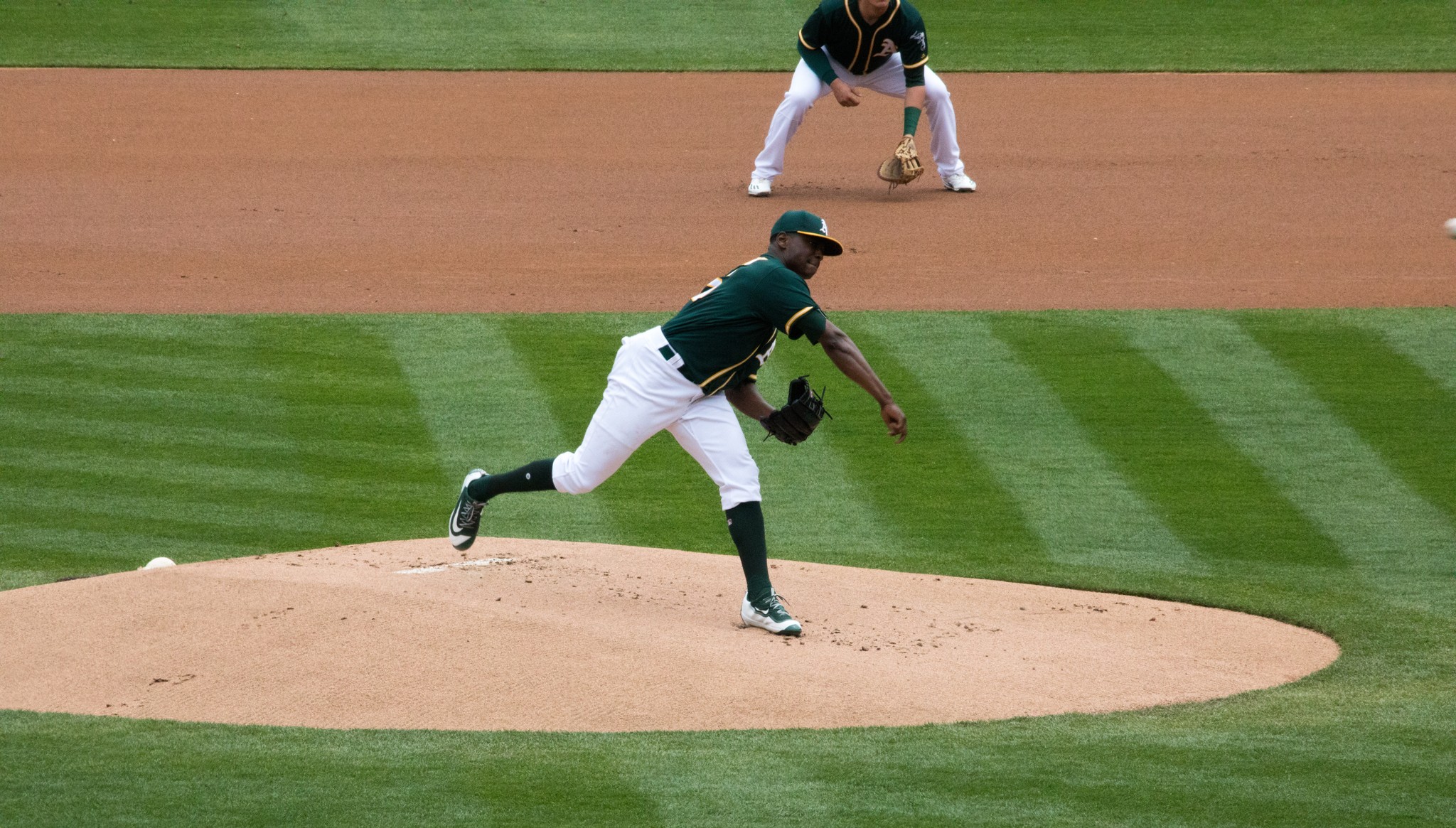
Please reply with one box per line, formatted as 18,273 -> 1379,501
748,179 -> 771,196
740,587 -> 802,635
942,174 -> 976,192
449,469 -> 490,551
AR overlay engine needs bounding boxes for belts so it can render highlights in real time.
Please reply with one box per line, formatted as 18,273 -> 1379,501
658,344 -> 699,385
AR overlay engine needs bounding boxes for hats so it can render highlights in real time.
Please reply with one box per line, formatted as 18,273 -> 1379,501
770,210 -> 843,256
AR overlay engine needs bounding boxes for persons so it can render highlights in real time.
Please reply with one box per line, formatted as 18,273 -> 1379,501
748,1 -> 976,196
448,210 -> 908,635
